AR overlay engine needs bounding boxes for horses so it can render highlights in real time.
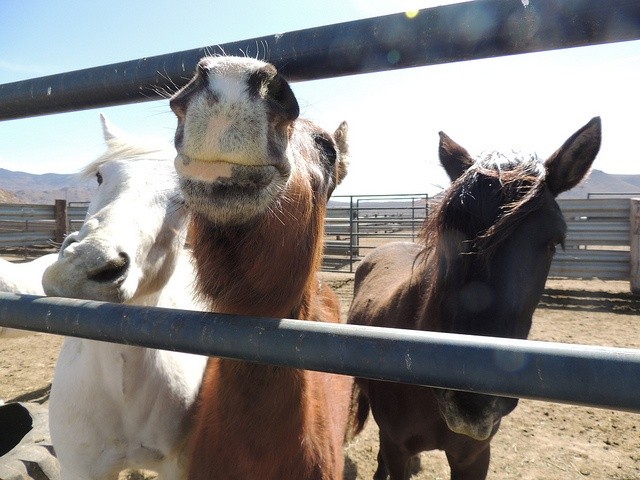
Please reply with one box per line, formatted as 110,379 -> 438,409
42,113 -> 212,480
147,38 -> 351,480
0,253 -> 60,336
341,115 -> 602,480
